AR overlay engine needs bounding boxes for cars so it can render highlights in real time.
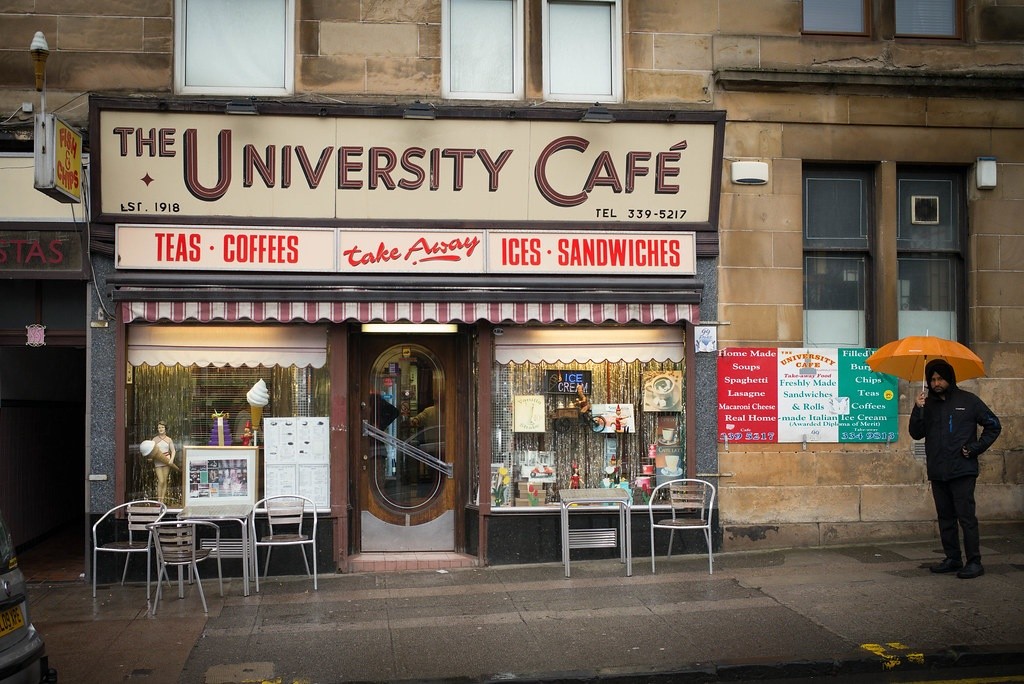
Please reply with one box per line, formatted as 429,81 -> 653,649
0,515 -> 59,684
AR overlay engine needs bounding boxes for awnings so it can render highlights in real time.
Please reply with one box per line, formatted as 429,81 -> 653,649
111,284 -> 702,328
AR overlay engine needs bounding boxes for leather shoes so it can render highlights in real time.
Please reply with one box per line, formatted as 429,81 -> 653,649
957,560 -> 984,579
930,557 -> 963,573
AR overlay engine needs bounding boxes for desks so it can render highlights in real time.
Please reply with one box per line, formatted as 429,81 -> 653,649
176,504 -> 255,600
558,488 -> 632,577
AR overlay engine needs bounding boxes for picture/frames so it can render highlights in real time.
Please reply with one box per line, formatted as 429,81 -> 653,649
181,446 -> 259,504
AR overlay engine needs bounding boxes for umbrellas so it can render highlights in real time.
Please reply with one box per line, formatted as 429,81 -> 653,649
864,328 -> 985,422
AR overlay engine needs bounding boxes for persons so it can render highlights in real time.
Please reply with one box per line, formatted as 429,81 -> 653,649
412,405 -> 436,444
908,358 -> 1001,578
146,420 -> 175,506
577,386 -> 591,426
569,467 -> 584,489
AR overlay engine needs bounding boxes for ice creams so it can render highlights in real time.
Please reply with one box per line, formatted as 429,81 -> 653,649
139,439 -> 179,470
246,378 -> 270,429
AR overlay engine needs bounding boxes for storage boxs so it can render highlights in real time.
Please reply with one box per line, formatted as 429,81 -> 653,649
515,497 -> 545,506
520,490 -> 546,499
519,482 -> 543,491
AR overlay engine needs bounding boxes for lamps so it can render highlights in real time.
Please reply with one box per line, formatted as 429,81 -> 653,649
975,156 -> 999,191
732,160 -> 769,186
31,29 -> 50,114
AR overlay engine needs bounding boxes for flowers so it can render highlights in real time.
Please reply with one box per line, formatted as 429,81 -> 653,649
493,467 -> 510,505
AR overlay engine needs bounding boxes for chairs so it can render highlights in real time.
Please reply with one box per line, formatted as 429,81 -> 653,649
92,501 -> 170,601
647,478 -> 716,575
253,494 -> 318,592
144,521 -> 224,615
